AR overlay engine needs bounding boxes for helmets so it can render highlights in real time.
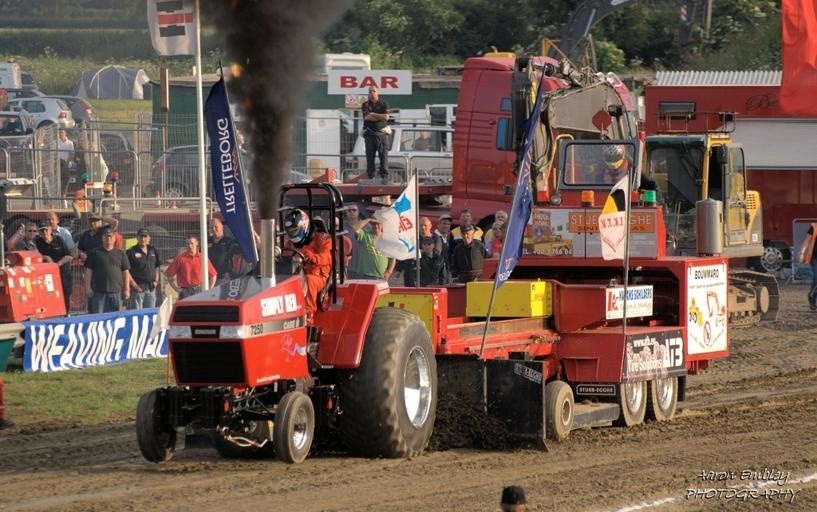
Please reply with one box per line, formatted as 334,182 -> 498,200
283,209 -> 311,248
601,144 -> 625,170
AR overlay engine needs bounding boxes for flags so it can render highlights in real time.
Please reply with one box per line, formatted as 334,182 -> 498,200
597,174 -> 628,261
146,0 -> 200,61
372,173 -> 421,260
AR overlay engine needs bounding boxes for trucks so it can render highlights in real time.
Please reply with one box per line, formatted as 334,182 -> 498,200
131,44 -> 643,253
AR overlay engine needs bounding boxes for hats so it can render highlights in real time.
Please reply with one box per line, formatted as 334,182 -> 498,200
103,229 -> 114,236
422,238 -> 434,244
138,227 -> 149,236
461,224 -> 473,232
38,222 -> 50,230
439,215 -> 452,222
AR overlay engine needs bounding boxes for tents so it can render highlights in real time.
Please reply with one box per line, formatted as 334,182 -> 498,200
68,65 -> 150,101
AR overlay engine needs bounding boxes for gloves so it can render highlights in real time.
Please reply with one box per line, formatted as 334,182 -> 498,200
303,250 -> 316,264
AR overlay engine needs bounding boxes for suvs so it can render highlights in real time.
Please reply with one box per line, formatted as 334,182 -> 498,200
350,122 -> 455,183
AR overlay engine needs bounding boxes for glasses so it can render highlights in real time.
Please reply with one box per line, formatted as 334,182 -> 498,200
347,208 -> 357,211
26,229 -> 37,232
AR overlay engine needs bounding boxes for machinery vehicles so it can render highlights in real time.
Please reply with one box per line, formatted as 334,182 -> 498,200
638,108 -> 781,334
130,183 -> 437,465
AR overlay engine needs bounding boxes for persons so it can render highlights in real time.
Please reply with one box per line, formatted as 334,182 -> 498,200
500,485 -> 528,512
590,145 -> 658,208
796,223 -> 816,308
72,139 -> 79,151
271,208 -> 331,334
410,130 -> 433,152
361,87 -> 393,180
0,375 -> 15,432
53,129 -> 74,208
72,135 -> 87,191
